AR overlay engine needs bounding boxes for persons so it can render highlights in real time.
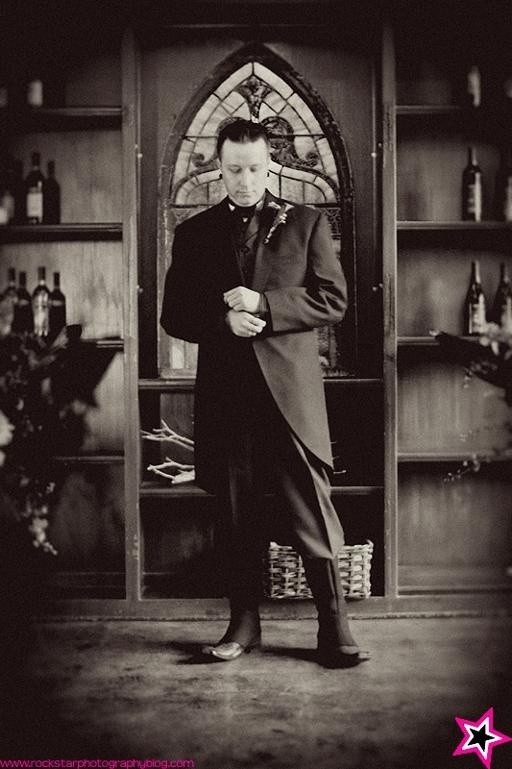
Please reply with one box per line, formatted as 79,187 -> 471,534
161,122 -> 369,666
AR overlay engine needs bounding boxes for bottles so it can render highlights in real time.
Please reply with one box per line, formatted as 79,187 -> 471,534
463,47 -> 512,342
0,38 -> 69,339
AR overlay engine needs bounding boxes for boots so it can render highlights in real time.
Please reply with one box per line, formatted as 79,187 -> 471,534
306,557 -> 361,667
198,569 -> 262,660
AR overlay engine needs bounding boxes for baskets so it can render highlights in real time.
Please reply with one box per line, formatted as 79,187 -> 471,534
263,538 -> 373,601
